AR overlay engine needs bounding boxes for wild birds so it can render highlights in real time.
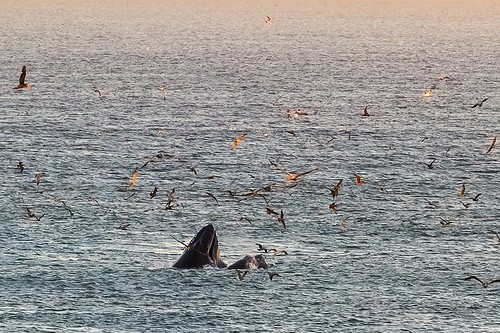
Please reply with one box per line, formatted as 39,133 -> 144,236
470,97 -> 490,109
360,106 -> 374,118
338,129 -> 351,141
486,136 -> 497,154
230,133 -> 248,151
156,85 -> 167,101
437,76 -> 451,84
11,65 -> 33,91
15,159 -> 82,223
234,268 -> 282,282
88,86 -> 102,96
223,151 -> 320,230
420,136 -> 434,143
462,275 -> 500,289
270,94 -> 309,138
256,243 -> 289,257
423,182 -> 483,227
445,145 -> 455,158
92,154 -> 219,216
112,219 -> 144,231
324,133 -> 338,145
322,173 -> 368,230
167,232 -> 208,257
421,159 -> 436,170
377,185 -> 389,195
264,15 -> 272,24
421,90 -> 434,96
484,229 -> 500,243
